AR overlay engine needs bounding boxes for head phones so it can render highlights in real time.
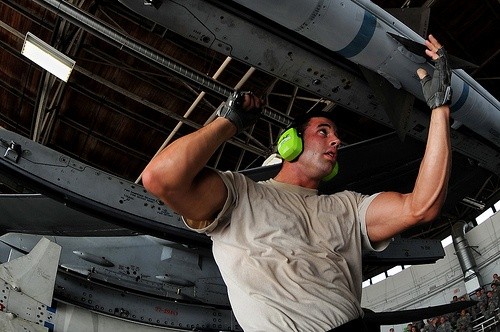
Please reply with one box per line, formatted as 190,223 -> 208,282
277,112 -> 339,182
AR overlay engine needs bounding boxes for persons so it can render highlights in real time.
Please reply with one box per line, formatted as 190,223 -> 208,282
142,34 -> 455,332
